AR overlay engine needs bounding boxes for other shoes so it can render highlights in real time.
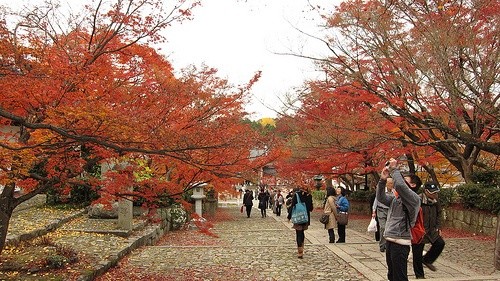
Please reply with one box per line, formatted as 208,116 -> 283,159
329,240 -> 334,243
416,276 -> 426,279
421,256 -> 436,271
336,239 -> 345,243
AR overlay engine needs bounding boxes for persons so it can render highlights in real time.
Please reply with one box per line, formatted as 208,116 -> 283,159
372,177 -> 396,252
412,180 -> 445,279
238,186 -> 295,221
375,157 -> 422,281
287,184 -> 314,258
323,186 -> 340,243
335,186 -> 351,243
370,185 -> 381,242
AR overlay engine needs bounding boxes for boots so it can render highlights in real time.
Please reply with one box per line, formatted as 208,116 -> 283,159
298,242 -> 304,258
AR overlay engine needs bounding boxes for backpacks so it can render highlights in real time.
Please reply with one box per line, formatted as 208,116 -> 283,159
402,199 -> 426,245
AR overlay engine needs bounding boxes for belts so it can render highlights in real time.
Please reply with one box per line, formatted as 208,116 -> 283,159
377,206 -> 389,210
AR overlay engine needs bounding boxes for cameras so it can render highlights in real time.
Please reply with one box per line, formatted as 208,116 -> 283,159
385,161 -> 390,166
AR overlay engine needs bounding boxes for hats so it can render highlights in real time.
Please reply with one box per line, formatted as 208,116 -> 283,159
423,181 -> 440,194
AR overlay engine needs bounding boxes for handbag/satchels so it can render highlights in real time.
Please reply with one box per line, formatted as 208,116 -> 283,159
292,193 -> 309,225
367,217 -> 378,232
320,214 -> 330,224
336,208 -> 348,225
